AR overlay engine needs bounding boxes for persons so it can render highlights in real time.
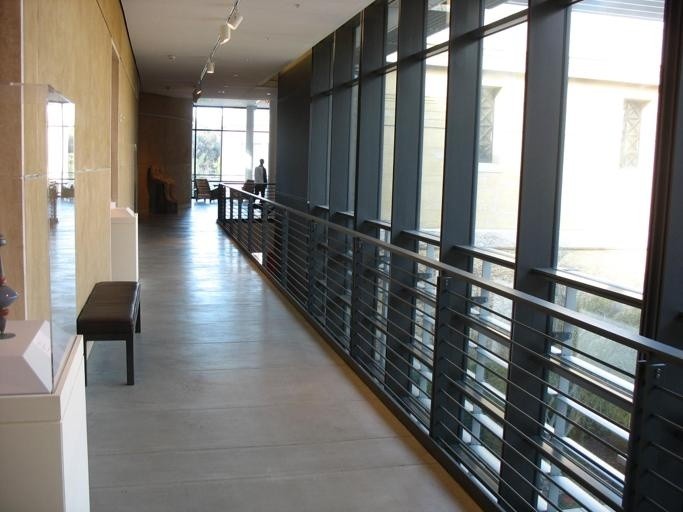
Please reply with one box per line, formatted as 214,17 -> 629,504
254,158 -> 267,197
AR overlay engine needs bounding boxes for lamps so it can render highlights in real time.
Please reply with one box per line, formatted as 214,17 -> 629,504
218,24 -> 230,43
227,5 -> 242,29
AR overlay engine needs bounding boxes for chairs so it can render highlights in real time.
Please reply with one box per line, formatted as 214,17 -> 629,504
195,178 -> 222,204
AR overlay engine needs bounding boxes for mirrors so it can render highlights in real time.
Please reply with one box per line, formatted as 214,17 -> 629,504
45,83 -> 78,393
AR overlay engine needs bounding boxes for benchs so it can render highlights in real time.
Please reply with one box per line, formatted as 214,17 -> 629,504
77,281 -> 141,386
